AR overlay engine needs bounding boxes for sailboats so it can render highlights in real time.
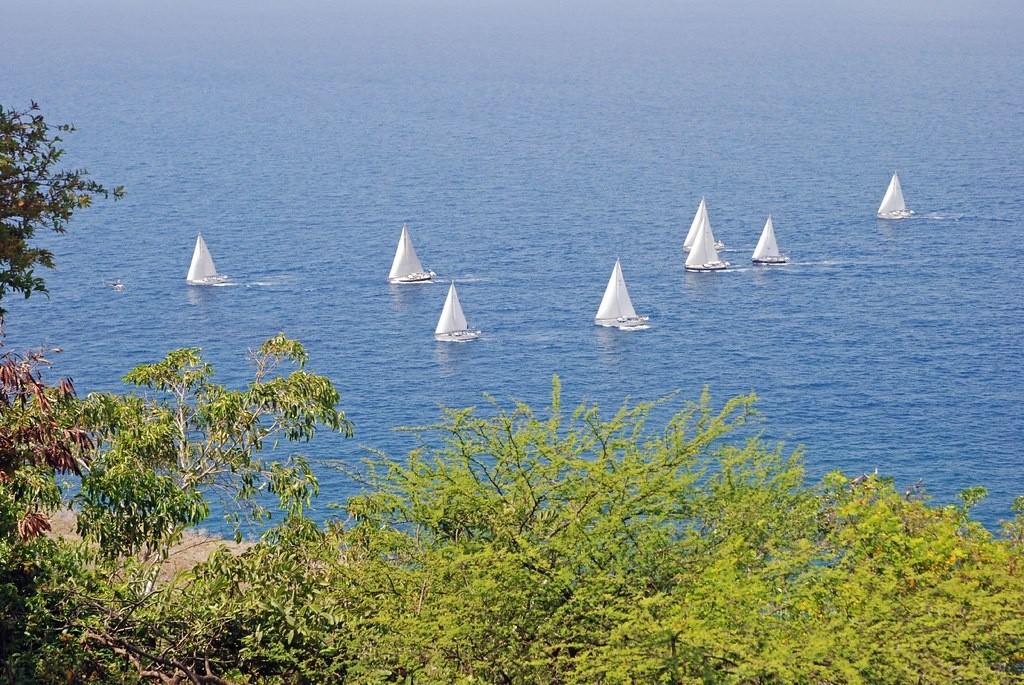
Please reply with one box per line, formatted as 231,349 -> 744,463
186,232 -> 227,285
386,222 -> 435,283
683,213 -> 730,270
431,279 -> 481,341
679,195 -> 726,250
876,172 -> 910,219
751,213 -> 790,264
594,257 -> 649,326
